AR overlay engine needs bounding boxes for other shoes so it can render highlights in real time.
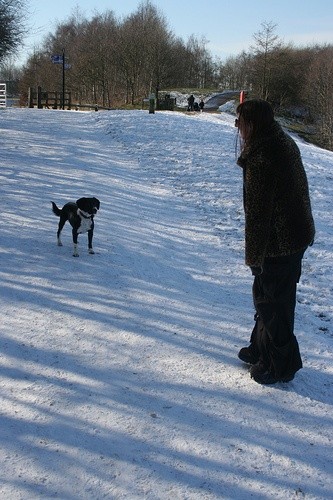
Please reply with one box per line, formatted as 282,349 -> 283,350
253,367 -> 295,385
238,347 -> 259,365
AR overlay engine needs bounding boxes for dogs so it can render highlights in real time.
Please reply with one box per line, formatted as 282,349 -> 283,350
50,197 -> 100,257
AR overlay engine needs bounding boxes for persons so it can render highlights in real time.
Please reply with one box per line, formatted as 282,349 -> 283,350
235,98 -> 317,386
193,101 -> 199,111
198,99 -> 204,109
186,95 -> 193,111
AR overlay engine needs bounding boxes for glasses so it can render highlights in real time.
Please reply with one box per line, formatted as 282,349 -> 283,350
234,119 -> 247,127
